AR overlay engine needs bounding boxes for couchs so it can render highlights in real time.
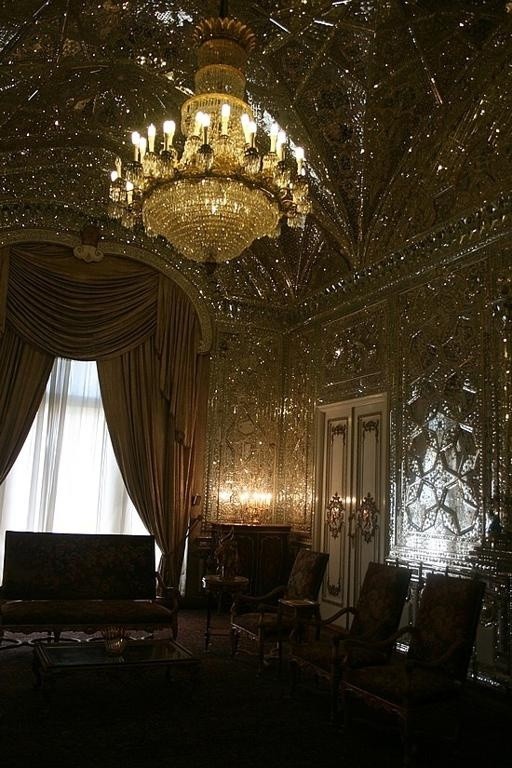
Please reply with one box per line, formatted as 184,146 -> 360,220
0,526 -> 182,651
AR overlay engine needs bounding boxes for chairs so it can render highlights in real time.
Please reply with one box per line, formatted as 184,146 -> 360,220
277,562 -> 411,706
226,541 -> 328,670
330,571 -> 487,767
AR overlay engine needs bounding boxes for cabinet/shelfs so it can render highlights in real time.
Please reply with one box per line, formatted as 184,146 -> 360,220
204,519 -> 295,602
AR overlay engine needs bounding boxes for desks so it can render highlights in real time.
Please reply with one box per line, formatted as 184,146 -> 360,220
199,573 -> 250,654
23,635 -> 192,745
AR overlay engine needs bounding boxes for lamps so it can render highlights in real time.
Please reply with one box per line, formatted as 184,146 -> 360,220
233,488 -> 278,526
102,11 -> 319,267
